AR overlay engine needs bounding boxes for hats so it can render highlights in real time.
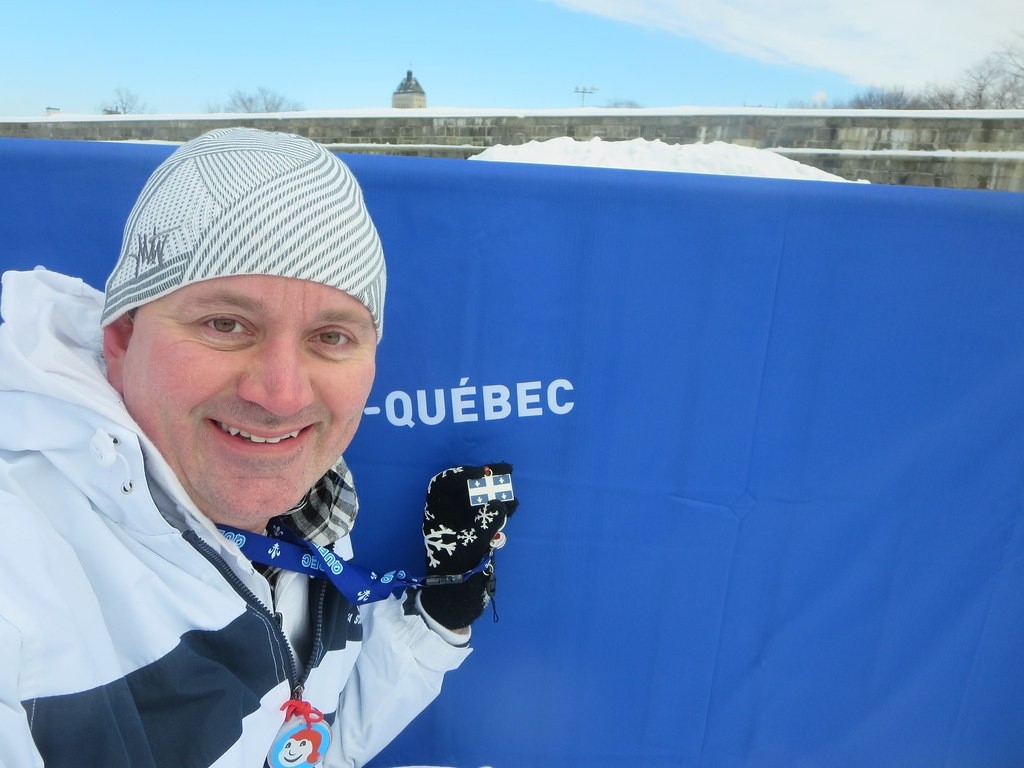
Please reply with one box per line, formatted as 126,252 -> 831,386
100,125 -> 388,345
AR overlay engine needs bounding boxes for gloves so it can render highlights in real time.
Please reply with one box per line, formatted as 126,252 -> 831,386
423,462 -> 519,629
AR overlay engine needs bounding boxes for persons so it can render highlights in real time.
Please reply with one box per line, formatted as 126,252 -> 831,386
0,126 -> 520,768
